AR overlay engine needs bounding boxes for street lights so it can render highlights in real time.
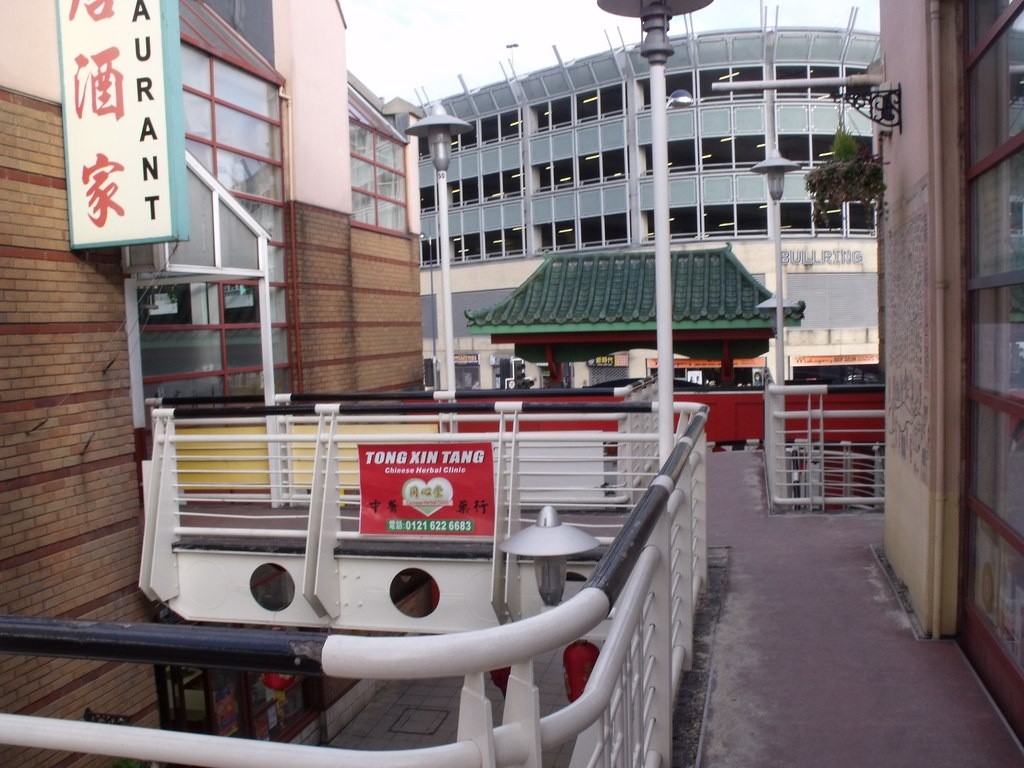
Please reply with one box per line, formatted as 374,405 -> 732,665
419,232 -> 437,390
404,103 -> 474,391
749,150 -> 802,386
597,1 -> 714,472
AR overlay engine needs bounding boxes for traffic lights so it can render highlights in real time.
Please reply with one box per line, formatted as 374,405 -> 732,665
513,359 -> 527,389
